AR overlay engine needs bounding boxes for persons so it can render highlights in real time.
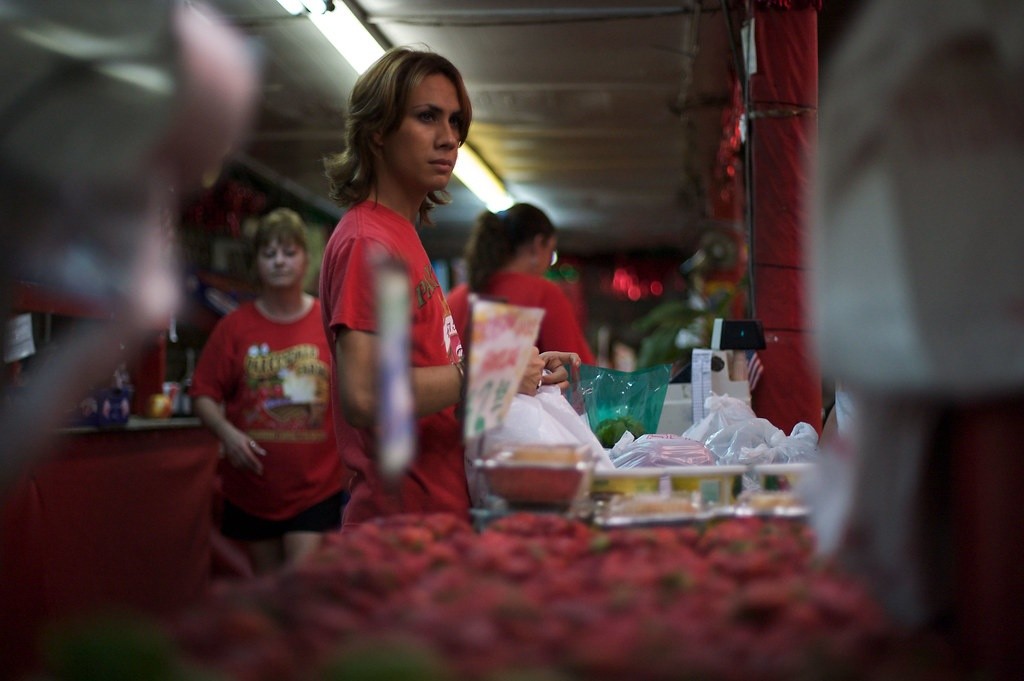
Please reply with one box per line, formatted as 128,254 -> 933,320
189,209 -> 350,574
317,47 -> 584,516
444,203 -> 594,416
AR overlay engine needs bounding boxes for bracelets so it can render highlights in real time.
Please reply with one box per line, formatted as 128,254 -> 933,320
455,361 -> 467,407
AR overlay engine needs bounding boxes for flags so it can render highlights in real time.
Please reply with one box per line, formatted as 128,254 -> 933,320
744,351 -> 763,391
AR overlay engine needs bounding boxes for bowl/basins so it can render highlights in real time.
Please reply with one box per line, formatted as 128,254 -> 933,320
468,438 -> 596,512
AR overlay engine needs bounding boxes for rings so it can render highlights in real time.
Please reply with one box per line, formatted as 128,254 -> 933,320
536,379 -> 542,389
